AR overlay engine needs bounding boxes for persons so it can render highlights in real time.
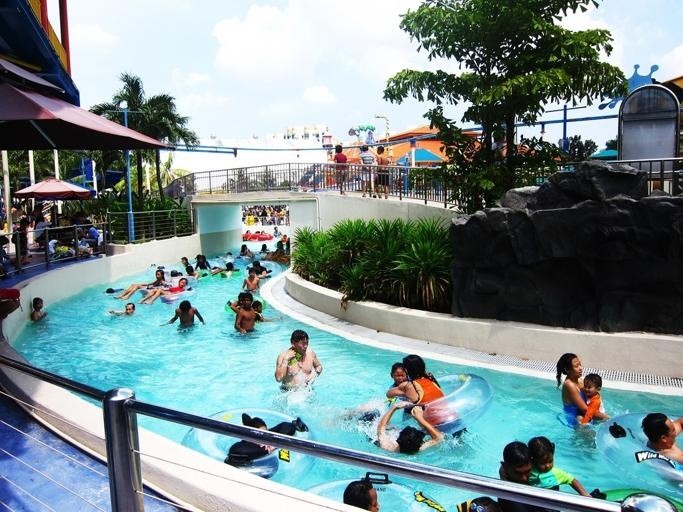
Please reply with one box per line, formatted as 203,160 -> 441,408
275,329 -> 323,394
330,400 -> 444,455
499,436 -> 592,512
388,362 -> 411,388
555,353 -> 610,447
0,202 -> 102,277
376,146 -> 391,200
360,144 -> 375,198
578,373 -> 606,425
105,203 -> 290,337
385,354 -> 465,441
241,413 -> 274,453
342,479 -> 381,512
333,145 -> 348,195
364,131 -> 375,146
641,410 -> 682,464
31,298 -> 47,321
497,441 -> 561,512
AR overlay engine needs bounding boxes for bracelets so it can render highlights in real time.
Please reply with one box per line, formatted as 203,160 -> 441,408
314,370 -> 320,375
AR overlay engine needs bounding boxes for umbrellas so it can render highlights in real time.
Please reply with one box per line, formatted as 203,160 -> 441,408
35,178 -> 95,217
0,59 -> 172,151
13,176 -> 91,239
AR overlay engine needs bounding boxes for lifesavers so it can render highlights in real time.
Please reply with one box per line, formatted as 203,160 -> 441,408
180,410 -> 314,485
385,375 -> 491,436
226,291 -> 266,316
161,267 -> 242,305
304,472 -> 448,511
595,409 -> 683,485
